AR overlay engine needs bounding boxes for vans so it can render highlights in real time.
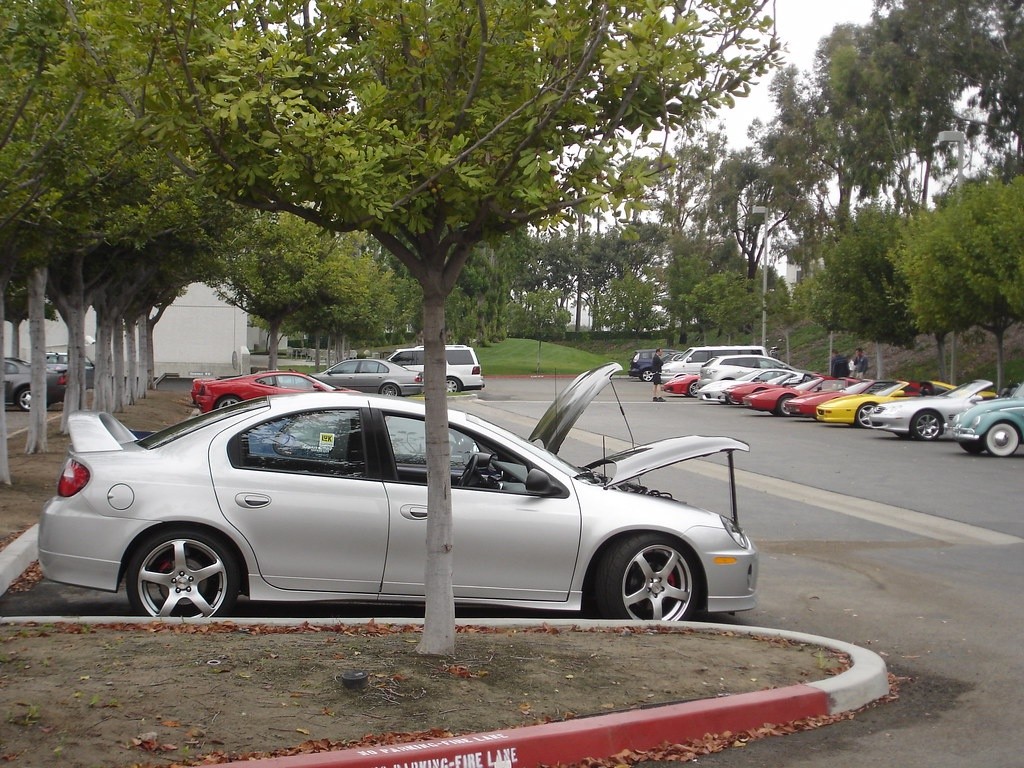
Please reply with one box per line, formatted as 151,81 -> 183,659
658,346 -> 768,383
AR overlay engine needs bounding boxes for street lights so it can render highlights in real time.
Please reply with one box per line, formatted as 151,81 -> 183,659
753,205 -> 768,350
938,130 -> 964,383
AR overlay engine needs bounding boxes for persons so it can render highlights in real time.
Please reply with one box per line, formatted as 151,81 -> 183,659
854,348 -> 868,378
773,348 -> 779,359
848,356 -> 856,377
831,350 -> 850,377
751,350 -> 760,368
651,348 -> 666,402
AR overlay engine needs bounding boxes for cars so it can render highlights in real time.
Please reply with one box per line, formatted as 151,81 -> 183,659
4,357 -> 67,413
943,382 -> 1024,457
190,368 -> 365,414
38,362 -> 761,621
309,357 -> 424,397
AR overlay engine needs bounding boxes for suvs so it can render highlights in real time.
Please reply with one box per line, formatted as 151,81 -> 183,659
628,349 -> 681,382
46,352 -> 95,391
378,345 -> 484,394
698,354 -> 820,389
660,352 -> 688,365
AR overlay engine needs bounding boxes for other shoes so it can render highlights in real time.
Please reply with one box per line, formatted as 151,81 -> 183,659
653,397 -> 658,401
657,397 -> 665,401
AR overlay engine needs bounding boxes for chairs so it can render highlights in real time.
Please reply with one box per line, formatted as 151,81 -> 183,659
1001,387 -> 1017,397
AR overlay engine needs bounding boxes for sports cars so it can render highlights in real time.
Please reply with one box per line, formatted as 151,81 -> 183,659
743,375 -> 841,417
695,369 -> 805,404
868,378 -> 1011,442
784,379 -> 904,420
663,373 -> 699,398
724,374 -> 804,406
815,379 -> 999,430
